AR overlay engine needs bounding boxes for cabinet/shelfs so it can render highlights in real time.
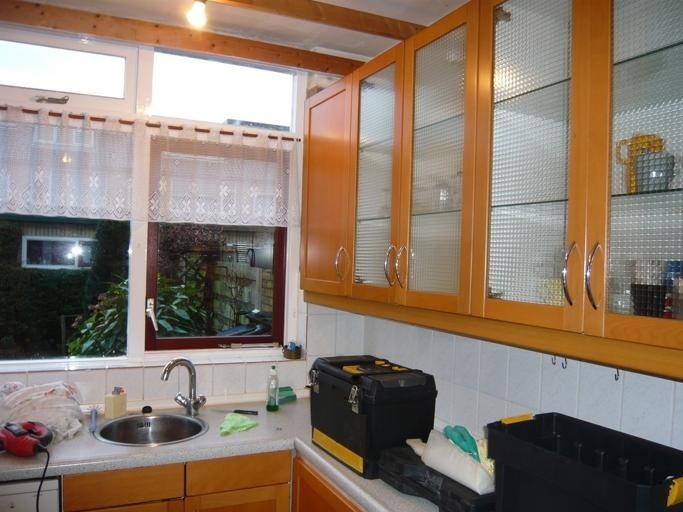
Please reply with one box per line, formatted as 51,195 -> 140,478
63,452 -> 292,512
294,457 -> 359,512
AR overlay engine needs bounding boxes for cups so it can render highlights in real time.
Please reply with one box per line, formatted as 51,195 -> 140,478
545,275 -> 564,306
287,341 -> 295,350
615,135 -> 675,193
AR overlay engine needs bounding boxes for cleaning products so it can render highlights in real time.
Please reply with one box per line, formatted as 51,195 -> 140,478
267,366 -> 280,411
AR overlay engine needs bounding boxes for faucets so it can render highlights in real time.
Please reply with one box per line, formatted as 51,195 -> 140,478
161,358 -> 196,399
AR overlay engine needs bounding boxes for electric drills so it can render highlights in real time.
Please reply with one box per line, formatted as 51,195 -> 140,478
0,421 -> 53,458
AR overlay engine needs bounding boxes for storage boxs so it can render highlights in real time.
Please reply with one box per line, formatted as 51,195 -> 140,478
309,356 -> 438,479
486,413 -> 682,508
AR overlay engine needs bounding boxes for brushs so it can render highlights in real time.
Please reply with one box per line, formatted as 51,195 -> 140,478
80,403 -> 104,433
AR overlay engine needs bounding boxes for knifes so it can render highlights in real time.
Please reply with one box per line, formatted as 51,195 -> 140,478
210,405 -> 258,415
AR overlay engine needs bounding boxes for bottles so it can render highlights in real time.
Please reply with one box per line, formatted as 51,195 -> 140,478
606,258 -> 683,320
432,167 -> 464,211
266,365 -> 282,412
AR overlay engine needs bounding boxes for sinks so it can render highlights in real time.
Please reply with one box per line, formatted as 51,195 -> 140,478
93,414 -> 209,448
